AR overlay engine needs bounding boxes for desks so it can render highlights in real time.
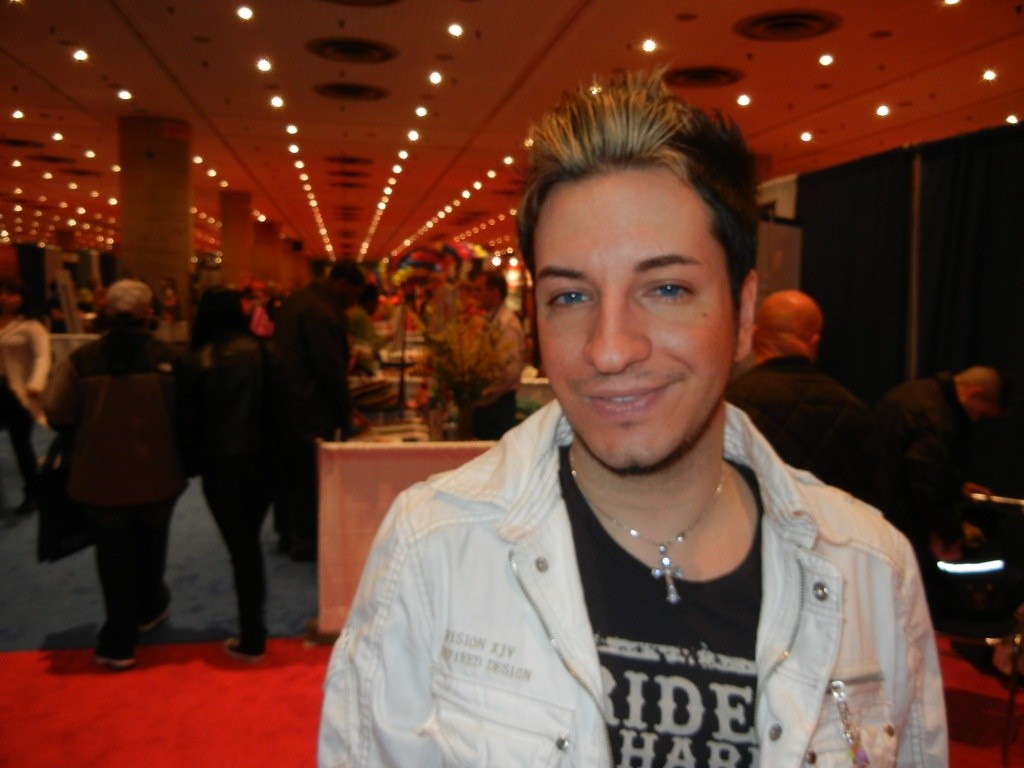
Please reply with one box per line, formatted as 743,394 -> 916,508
317,373 -> 554,638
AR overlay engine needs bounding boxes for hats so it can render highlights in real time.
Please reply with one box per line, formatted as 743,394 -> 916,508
101,278 -> 153,320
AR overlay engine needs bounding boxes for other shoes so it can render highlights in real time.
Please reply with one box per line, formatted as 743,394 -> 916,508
226,638 -> 266,663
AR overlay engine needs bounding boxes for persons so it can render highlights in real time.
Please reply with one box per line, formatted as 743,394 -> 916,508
317,76 -> 950,768
728,290 -> 1000,629
0,256 -> 525,668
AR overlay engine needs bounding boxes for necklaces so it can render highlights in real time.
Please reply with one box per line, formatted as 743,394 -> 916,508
569,450 -> 724,604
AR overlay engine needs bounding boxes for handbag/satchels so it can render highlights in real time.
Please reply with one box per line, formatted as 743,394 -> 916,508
35,439 -> 93,564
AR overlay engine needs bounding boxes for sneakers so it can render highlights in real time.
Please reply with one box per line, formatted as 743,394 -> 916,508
93,640 -> 137,668
140,601 -> 172,632
